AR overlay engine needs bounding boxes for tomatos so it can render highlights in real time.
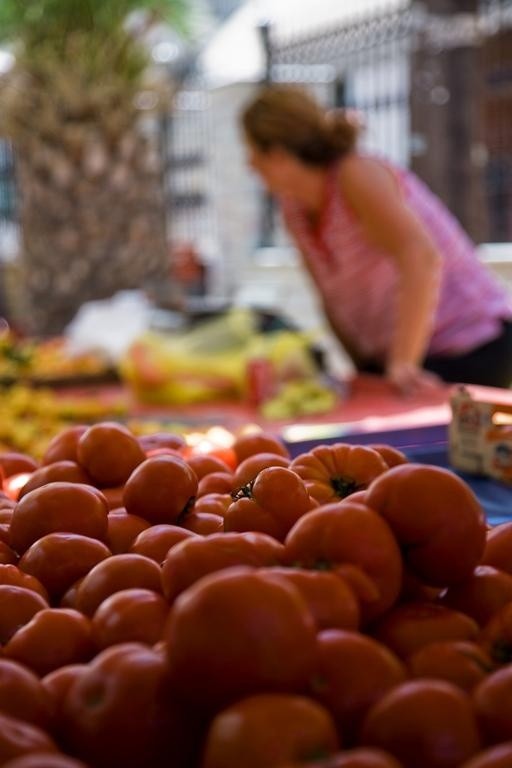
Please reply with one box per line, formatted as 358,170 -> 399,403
0,422 -> 512,768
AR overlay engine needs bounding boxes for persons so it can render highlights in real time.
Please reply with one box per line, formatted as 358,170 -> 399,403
236,83 -> 511,397
